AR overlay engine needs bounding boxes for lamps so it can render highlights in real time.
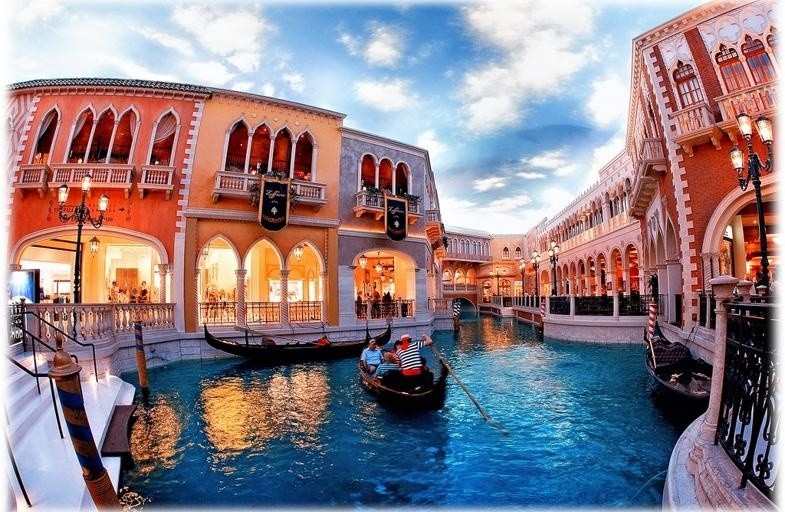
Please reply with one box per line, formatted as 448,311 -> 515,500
293,245 -> 303,263
375,252 -> 383,276
358,254 -> 367,269
154,263 -> 159,273
88,234 -> 100,259
201,242 -> 211,261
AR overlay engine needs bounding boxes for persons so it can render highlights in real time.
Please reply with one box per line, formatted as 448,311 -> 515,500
390,340 -> 402,354
374,351 -> 400,376
317,334 -> 332,346
381,332 -> 433,391
360,339 -> 384,374
355,285 -> 392,320
753,270 -> 764,293
110,280 -> 150,312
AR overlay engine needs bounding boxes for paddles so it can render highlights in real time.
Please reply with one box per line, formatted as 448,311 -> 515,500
429,342 -> 511,437
233,326 -> 326,347
646,323 -> 658,369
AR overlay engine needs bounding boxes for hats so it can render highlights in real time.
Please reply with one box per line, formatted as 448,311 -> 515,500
400,334 -> 412,341
369,339 -> 376,344
394,340 -> 402,348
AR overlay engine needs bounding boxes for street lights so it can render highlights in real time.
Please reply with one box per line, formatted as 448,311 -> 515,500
518,257 -> 527,296
57,171 -> 112,342
496,266 -> 500,296
546,239 -> 561,296
728,110 -> 775,302
530,249 -> 541,296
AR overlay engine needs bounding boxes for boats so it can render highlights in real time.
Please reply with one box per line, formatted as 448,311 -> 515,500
643,316 -> 713,401
203,323 -> 394,364
359,319 -> 448,414
